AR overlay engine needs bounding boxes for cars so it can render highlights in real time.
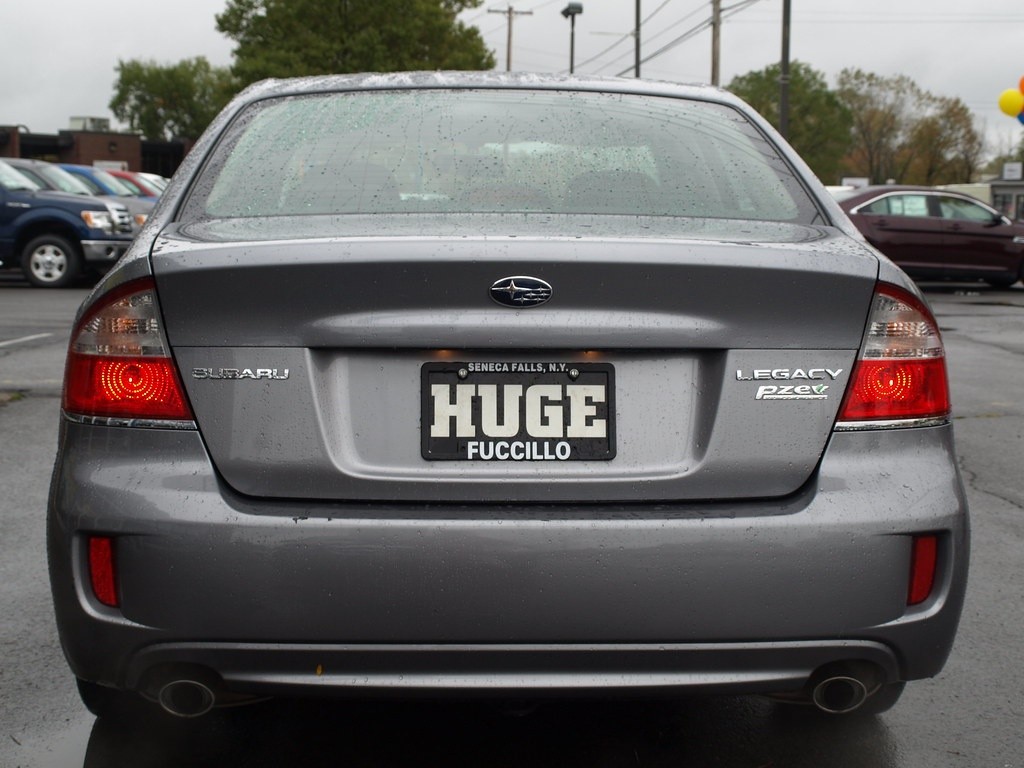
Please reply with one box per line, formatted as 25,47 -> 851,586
834,185 -> 1024,289
0,158 -> 181,288
41,71 -> 974,735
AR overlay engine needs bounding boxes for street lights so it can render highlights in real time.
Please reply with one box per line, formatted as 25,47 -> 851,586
563,1 -> 585,76
